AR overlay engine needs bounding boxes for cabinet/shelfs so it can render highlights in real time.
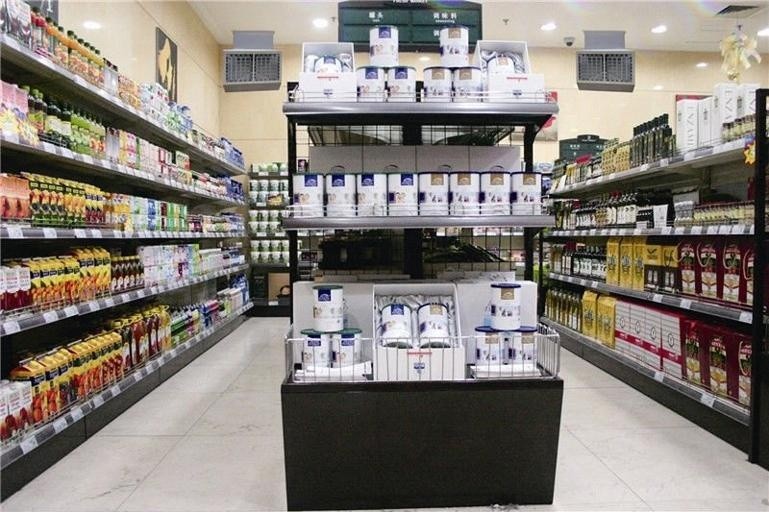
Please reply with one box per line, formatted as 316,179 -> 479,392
540,88 -> 769,474
249,163 -> 290,317
0,1 -> 247,512
281,89 -> 561,511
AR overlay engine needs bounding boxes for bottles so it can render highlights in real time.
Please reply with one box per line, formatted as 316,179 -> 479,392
23,84 -> 106,160
102,190 -> 128,227
632,114 -> 672,166
171,274 -> 250,348
107,302 -> 172,370
552,157 -> 565,177
722,110 -> 769,143
545,286 -> 582,332
563,188 -> 652,232
111,256 -> 145,291
31,6 -> 106,88
566,159 -> 601,184
601,137 -> 632,172
673,197 -> 769,227
551,243 -> 608,280
